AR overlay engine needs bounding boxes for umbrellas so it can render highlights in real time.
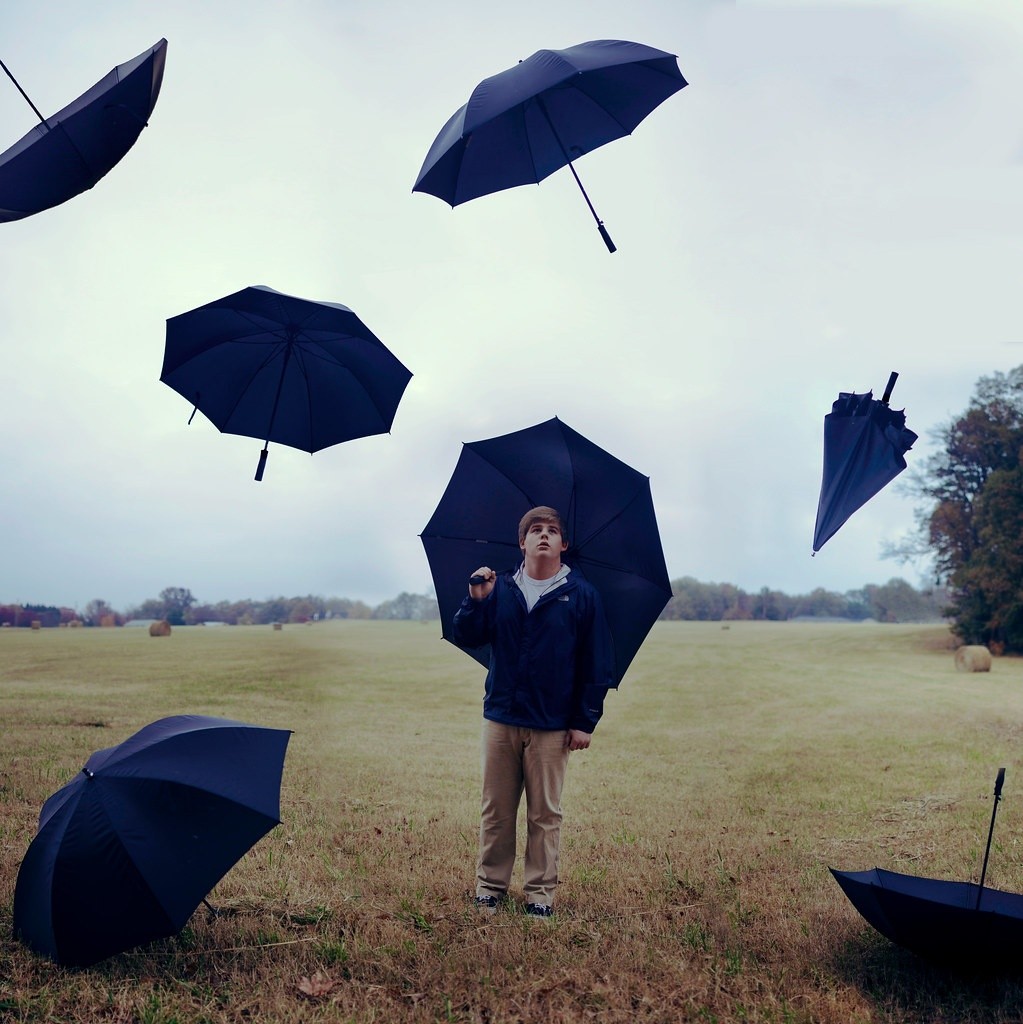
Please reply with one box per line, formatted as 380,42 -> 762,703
810,370 -> 920,557
159,285 -> 413,482
416,415 -> 674,692
0,38 -> 168,224
13,715 -> 296,967
826,767 -> 1023,988
411,40 -> 688,254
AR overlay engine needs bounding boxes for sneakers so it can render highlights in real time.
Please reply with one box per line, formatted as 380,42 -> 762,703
526,903 -> 553,919
476,895 -> 498,911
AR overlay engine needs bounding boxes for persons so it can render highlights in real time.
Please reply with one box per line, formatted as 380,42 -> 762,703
452,506 -> 605,916
